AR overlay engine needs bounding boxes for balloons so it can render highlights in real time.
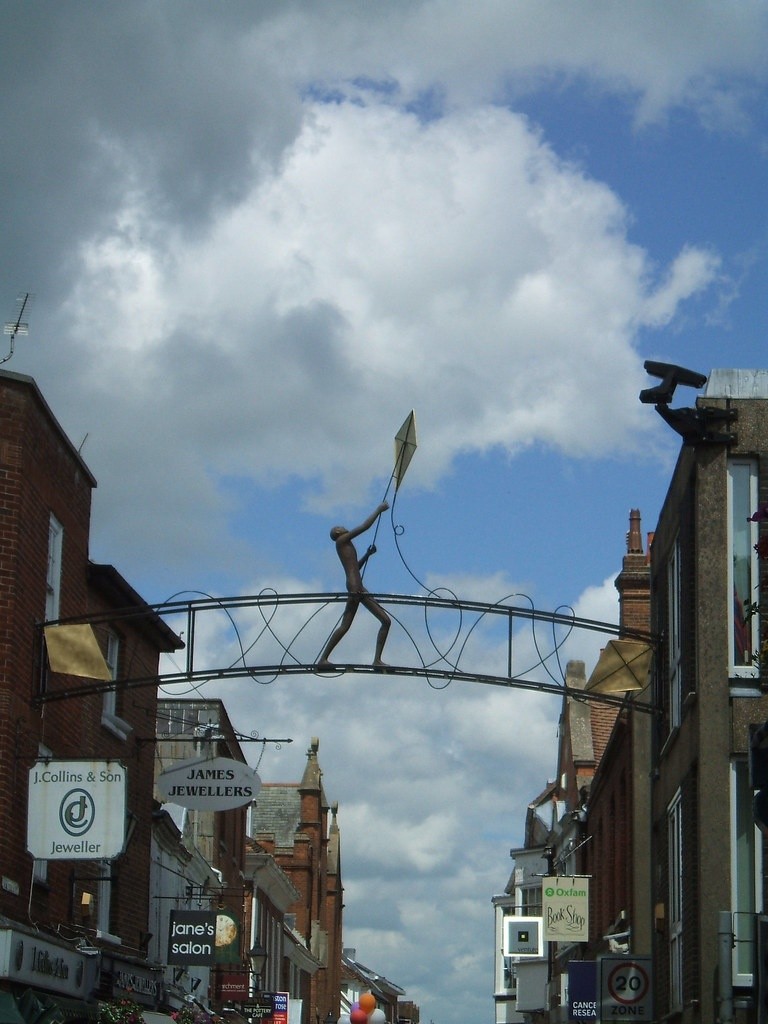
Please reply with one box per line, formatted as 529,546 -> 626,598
336,992 -> 385,1024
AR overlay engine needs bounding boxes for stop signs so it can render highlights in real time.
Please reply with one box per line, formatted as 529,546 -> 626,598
608,963 -> 650,1005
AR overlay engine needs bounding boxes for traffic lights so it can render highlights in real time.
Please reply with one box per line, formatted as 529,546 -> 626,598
749,720 -> 768,793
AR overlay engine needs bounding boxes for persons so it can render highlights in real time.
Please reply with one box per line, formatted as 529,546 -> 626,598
316,500 -> 392,666
